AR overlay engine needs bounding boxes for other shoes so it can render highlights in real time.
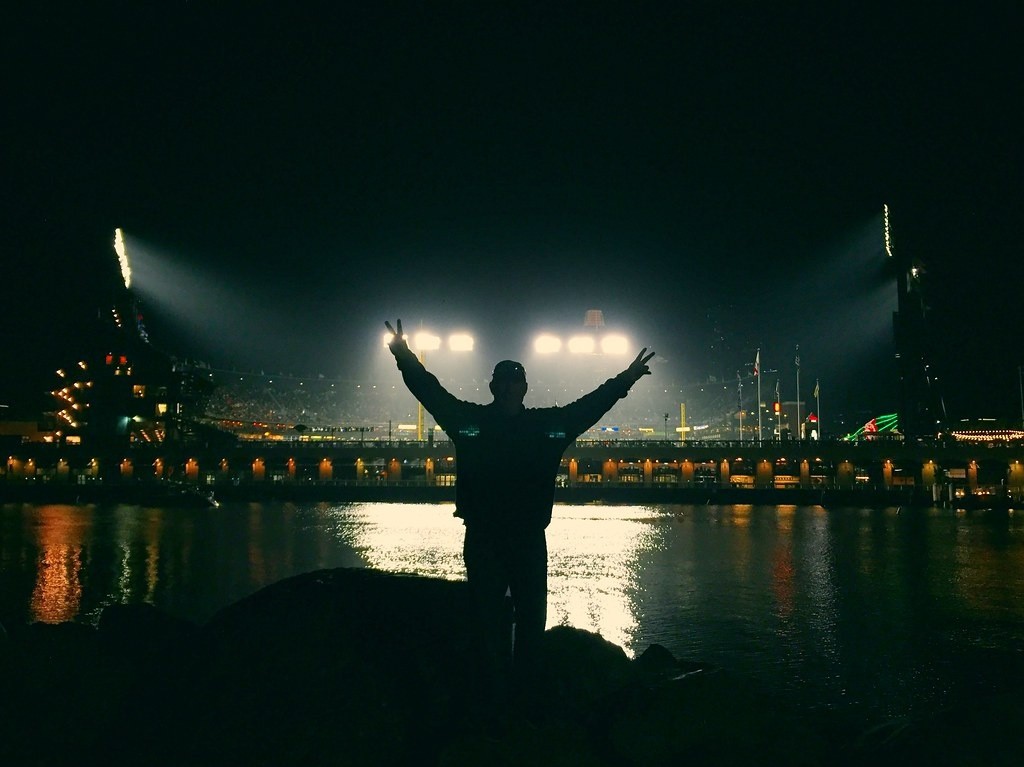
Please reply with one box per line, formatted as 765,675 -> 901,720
487,716 -> 507,741
517,696 -> 546,732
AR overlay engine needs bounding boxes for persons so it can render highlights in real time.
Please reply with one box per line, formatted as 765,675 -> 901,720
385,319 -> 657,742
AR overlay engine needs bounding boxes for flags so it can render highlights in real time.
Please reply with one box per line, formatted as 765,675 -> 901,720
734,379 -> 742,411
792,349 -> 802,374
752,352 -> 760,377
814,382 -> 820,398
775,380 -> 780,396
808,411 -> 819,423
